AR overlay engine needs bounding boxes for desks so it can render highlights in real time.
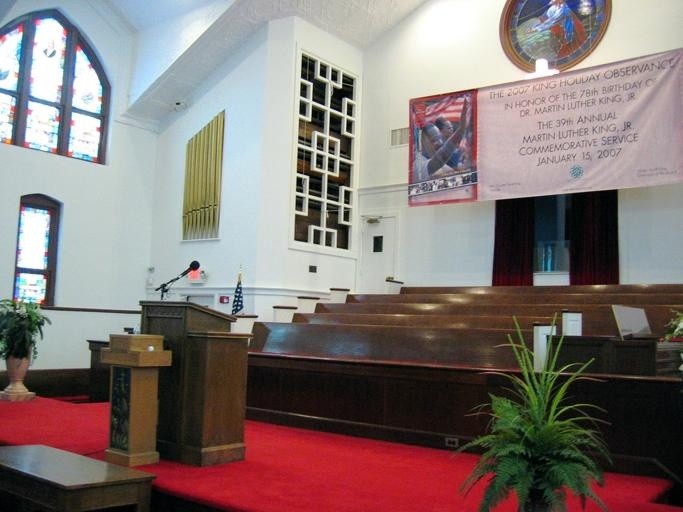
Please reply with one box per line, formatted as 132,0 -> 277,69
0,444 -> 157,511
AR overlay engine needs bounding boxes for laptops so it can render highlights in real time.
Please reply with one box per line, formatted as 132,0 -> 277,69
611,304 -> 652,341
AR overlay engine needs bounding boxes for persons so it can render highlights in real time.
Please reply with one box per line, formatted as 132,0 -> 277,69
524,0 -> 587,59
412,94 -> 471,183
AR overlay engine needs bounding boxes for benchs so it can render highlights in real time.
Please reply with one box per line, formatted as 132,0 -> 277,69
247,284 -> 683,477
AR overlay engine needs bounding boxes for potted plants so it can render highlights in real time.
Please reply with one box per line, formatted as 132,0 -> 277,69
0,296 -> 51,402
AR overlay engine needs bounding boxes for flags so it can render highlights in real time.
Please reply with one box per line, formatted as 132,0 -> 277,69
231,272 -> 243,315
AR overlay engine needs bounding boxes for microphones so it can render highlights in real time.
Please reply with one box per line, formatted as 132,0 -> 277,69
178,260 -> 200,279
